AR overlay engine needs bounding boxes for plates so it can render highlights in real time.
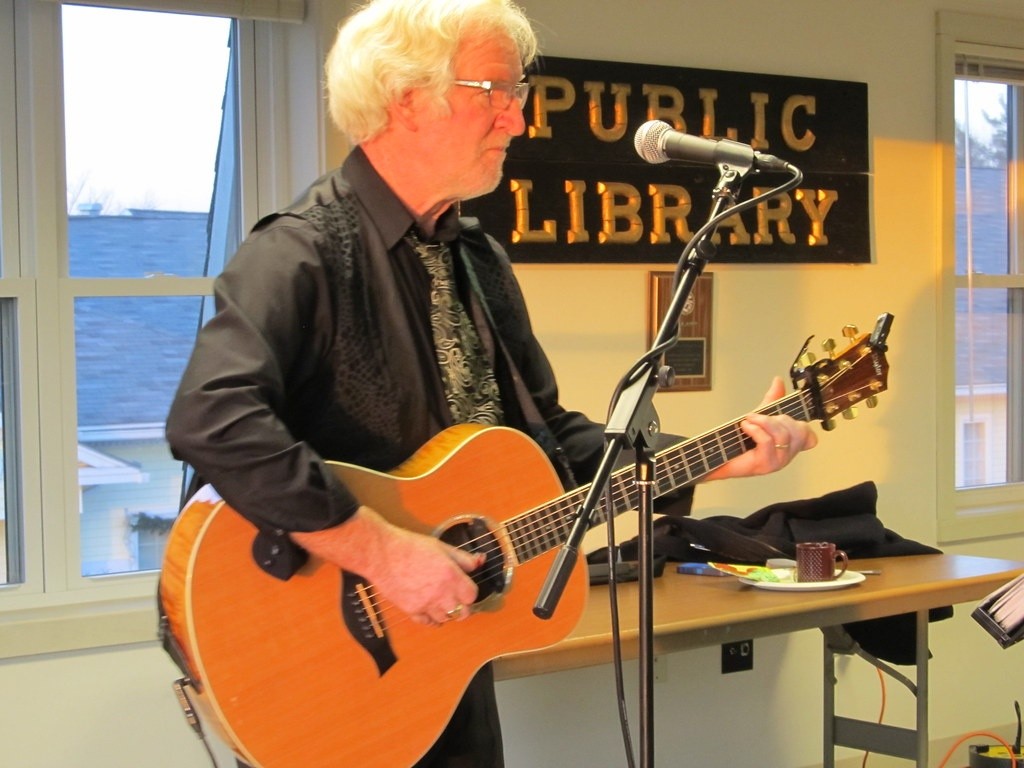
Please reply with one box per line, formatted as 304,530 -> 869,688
740,566 -> 866,590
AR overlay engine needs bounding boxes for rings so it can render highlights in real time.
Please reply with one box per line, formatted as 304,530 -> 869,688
773,443 -> 790,449
445,604 -> 463,620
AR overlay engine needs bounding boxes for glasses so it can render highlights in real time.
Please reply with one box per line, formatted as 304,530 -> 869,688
450,77 -> 530,111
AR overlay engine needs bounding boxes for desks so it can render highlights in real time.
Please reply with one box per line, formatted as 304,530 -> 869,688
492,552 -> 1024,767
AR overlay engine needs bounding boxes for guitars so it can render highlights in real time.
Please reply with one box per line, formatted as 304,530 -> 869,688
156,311 -> 896,767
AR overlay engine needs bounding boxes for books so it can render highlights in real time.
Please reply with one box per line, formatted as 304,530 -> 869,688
977,572 -> 1024,634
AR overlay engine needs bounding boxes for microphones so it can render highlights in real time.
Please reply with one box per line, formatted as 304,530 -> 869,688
635,120 -> 791,178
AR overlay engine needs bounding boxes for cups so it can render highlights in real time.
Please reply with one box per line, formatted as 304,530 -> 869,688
797,542 -> 849,584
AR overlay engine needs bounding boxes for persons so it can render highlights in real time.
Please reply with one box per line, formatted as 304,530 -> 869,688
164,0 -> 820,767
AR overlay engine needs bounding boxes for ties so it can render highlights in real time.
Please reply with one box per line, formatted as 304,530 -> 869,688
403,229 -> 507,427
